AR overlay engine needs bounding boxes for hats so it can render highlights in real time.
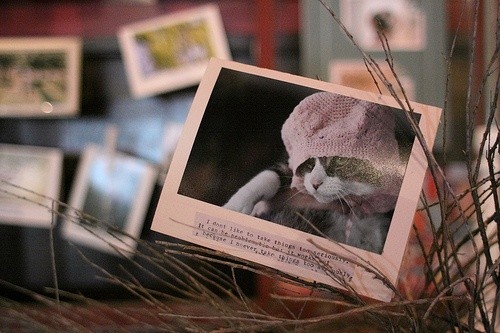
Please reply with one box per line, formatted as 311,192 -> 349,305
278,91 -> 402,193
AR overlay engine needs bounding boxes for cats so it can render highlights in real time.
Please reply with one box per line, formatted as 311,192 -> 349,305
221,155 -> 378,216
